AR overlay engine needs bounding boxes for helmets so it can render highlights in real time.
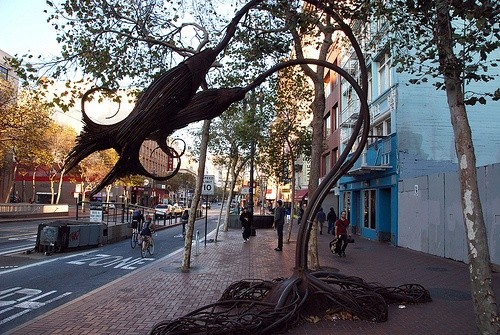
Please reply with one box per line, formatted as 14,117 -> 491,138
146,215 -> 152,220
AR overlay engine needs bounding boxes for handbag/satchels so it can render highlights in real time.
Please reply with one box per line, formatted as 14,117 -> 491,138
348,236 -> 355,243
329,238 -> 340,254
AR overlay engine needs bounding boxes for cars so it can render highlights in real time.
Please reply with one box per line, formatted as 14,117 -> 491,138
155,202 -> 221,220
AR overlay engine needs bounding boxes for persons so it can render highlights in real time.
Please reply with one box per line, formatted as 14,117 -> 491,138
131,207 -> 145,244
286,207 -> 290,224
296,209 -> 304,225
239,207 -> 252,243
316,206 -> 336,235
336,211 -> 349,258
274,199 -> 286,251
182,210 -> 189,234
140,217 -> 155,251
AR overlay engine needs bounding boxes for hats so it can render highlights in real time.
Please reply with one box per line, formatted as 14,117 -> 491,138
277,200 -> 282,203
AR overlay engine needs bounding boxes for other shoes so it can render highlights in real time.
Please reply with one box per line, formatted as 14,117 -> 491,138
274,247 -> 282,251
337,253 -> 346,257
244,239 -> 249,242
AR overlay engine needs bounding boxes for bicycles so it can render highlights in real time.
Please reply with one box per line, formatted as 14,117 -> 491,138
140,231 -> 154,258
130,223 -> 142,249
